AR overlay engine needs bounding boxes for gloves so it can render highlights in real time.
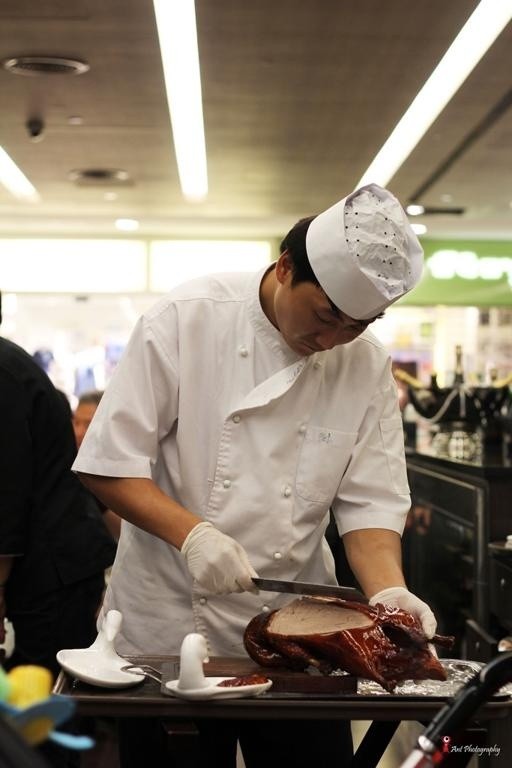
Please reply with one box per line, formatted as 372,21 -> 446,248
368,587 -> 439,661
181,520 -> 260,598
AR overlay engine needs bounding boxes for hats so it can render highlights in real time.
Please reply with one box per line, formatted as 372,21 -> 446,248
306,182 -> 425,322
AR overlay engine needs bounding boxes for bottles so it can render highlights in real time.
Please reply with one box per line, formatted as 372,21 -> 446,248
444,420 -> 475,460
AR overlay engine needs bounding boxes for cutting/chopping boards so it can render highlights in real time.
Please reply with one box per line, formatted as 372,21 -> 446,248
193,656 -> 359,693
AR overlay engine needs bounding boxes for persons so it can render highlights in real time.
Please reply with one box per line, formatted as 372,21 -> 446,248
70,184 -> 440,768
1,333 -> 115,649
71,389 -> 121,546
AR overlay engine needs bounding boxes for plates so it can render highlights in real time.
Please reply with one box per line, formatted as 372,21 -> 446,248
57,610 -> 148,687
166,632 -> 275,697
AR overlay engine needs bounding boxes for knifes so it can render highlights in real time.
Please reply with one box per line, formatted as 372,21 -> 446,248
245,576 -> 367,604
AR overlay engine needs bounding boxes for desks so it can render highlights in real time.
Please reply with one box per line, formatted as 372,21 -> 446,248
56,647 -> 511,731
403,437 -> 512,636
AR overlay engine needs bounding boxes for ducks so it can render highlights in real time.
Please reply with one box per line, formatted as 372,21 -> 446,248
242,595 -> 457,695
